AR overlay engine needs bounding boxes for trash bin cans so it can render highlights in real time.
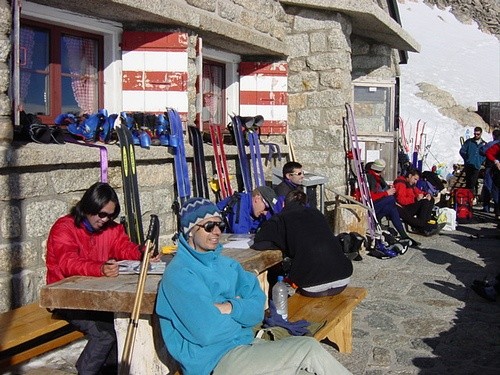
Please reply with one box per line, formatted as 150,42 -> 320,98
271,166 -> 329,215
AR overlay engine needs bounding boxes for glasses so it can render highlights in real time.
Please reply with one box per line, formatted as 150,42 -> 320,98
98,212 -> 114,220
256,194 -> 271,211
288,170 -> 305,176
474,133 -> 481,136
196,221 -> 226,233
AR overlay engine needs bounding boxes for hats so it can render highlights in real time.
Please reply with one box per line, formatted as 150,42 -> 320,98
256,186 -> 279,217
371,158 -> 386,171
179,197 -> 223,235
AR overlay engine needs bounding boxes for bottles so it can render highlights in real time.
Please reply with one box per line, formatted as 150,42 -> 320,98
273,276 -> 288,322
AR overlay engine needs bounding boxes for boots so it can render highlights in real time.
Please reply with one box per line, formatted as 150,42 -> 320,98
404,236 -> 418,246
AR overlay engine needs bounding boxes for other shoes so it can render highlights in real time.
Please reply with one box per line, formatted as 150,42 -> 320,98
345,252 -> 358,261
483,205 -> 489,212
424,222 -> 446,235
495,214 -> 500,225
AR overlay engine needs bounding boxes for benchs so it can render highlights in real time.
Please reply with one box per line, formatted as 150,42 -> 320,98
0,303 -> 85,370
174,287 -> 367,375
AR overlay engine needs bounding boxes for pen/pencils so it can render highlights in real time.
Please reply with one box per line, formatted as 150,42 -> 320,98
105,263 -> 128,267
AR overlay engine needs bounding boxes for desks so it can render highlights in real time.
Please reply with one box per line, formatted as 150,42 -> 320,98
39,234 -> 283,375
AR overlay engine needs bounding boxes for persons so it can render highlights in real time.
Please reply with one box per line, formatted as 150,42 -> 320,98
255,189 -> 353,298
481,129 -> 500,219
46,182 -> 156,375
365,162 -> 446,237
393,167 -> 435,234
358,160 -> 421,246
273,162 -> 308,196
460,127 -> 486,200
156,198 -> 352,375
216,186 -> 278,234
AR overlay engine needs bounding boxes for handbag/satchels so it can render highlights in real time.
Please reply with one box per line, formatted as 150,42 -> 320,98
253,324 -> 292,341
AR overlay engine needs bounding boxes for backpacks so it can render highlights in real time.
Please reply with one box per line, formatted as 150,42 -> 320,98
454,187 -> 474,223
